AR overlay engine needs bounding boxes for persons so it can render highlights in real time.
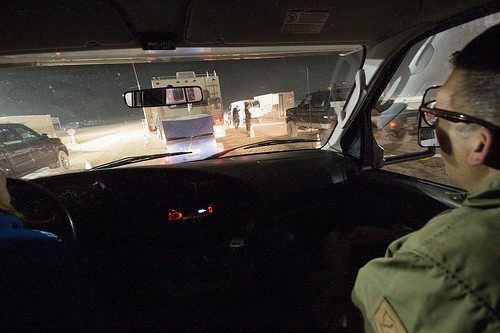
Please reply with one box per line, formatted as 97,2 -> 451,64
245,103 -> 252,137
233,105 -> 241,129
352,23 -> 500,333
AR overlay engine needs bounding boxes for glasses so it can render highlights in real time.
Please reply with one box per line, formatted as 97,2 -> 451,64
419,100 -> 500,133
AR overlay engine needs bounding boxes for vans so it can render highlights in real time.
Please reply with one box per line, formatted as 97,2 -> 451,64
229,99 -> 264,122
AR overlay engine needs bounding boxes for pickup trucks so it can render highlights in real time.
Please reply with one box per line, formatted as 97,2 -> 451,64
286,87 -> 408,141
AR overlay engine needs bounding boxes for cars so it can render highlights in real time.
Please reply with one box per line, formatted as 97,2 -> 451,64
0,122 -> 70,179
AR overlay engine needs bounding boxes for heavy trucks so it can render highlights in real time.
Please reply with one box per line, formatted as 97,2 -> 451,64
137,70 -> 226,142
